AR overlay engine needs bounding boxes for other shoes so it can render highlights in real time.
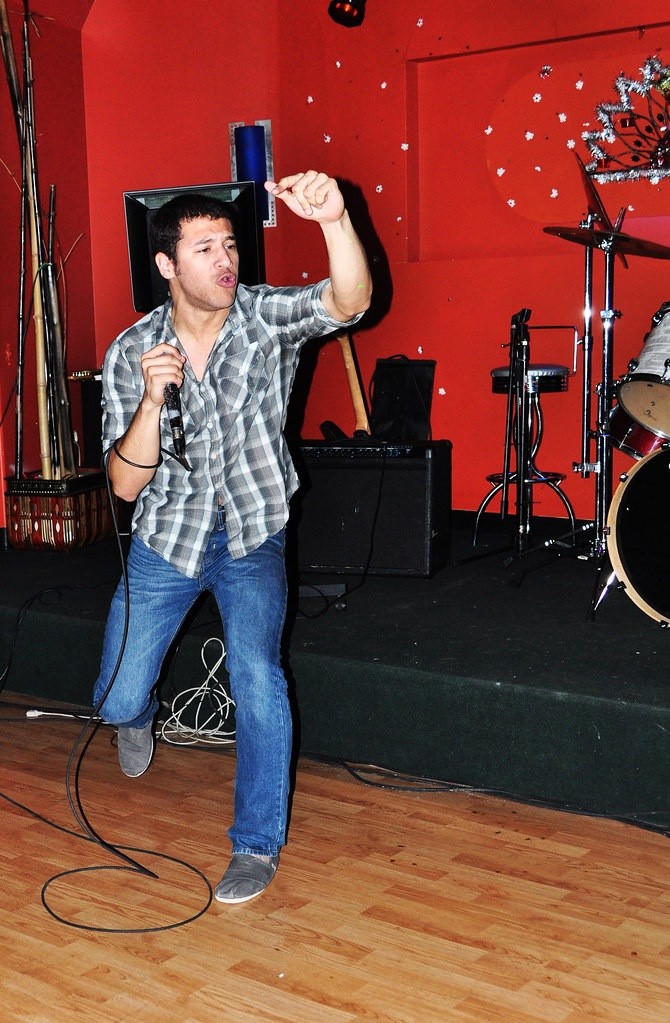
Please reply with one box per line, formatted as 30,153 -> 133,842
118,715 -> 154,777
215,854 -> 280,903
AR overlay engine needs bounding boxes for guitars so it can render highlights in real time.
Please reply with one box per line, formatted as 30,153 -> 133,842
317,328 -> 404,443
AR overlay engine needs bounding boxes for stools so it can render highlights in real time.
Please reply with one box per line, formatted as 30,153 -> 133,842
473,363 -> 576,550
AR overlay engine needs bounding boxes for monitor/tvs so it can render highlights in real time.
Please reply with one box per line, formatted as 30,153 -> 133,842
123,181 -> 263,315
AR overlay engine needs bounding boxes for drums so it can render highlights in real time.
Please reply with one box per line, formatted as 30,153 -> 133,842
606,441 -> 670,626
618,302 -> 669,440
491,364 -> 570,394
608,404 -> 669,463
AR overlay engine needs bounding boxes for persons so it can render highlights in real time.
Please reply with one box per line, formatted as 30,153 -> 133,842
93,168 -> 372,904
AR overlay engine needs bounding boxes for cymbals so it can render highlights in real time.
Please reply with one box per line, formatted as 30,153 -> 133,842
571,149 -> 629,269
542,224 -> 670,260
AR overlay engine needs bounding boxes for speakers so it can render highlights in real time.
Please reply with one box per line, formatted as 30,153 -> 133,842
279,439 -> 452,576
371,359 -> 436,443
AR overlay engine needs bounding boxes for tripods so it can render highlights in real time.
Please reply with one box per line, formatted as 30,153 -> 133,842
501,213 -> 621,619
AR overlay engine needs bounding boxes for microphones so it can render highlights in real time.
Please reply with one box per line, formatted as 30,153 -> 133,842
163,384 -> 185,458
